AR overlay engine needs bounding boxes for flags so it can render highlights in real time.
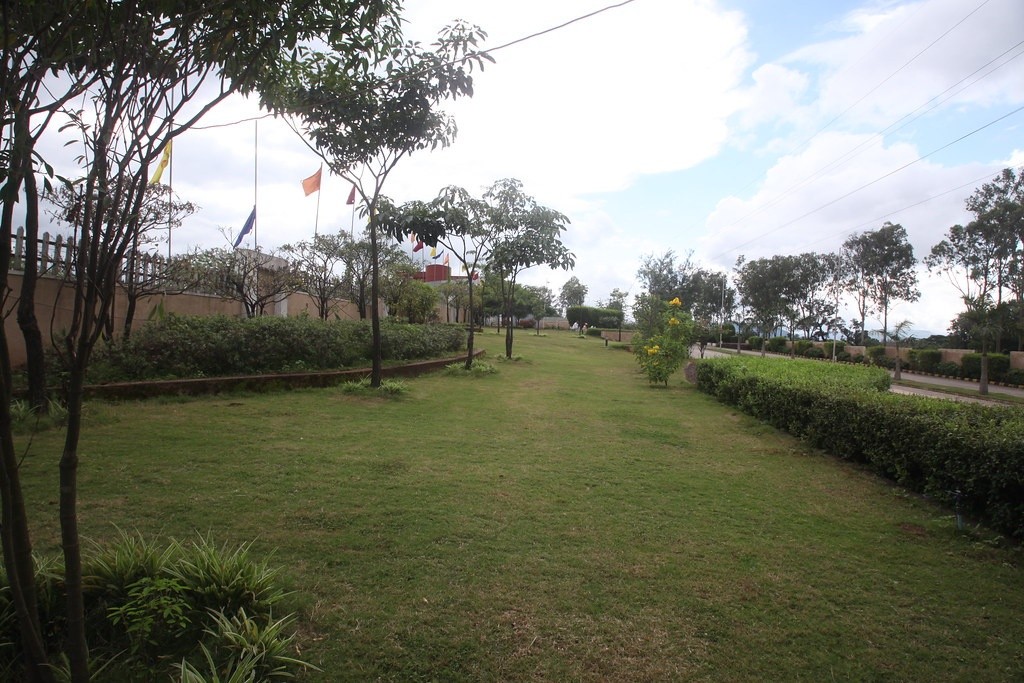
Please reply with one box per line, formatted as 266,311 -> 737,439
462,264 -> 470,272
413,240 -> 423,252
301,166 -> 321,195
430,248 -> 436,256
444,256 -> 448,263
432,251 -> 443,259
473,273 -> 478,280
347,185 -> 356,203
368,210 -> 376,222
410,232 -> 414,242
150,140 -> 172,185
232,208 -> 255,248
